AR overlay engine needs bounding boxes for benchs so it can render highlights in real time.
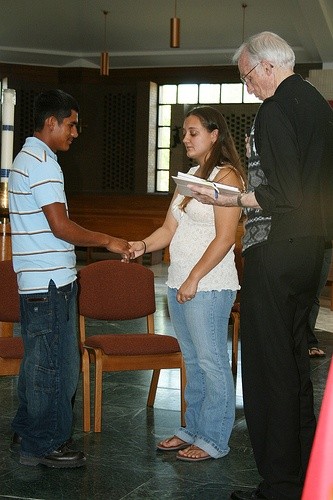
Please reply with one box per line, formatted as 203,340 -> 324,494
68,195 -> 333,311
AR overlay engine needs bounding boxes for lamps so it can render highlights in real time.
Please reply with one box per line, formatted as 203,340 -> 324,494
101,10 -> 109,77
169,0 -> 180,48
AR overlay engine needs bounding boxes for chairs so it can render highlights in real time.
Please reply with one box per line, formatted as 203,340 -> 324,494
77,260 -> 190,432
0,259 -> 90,433
164,248 -> 245,383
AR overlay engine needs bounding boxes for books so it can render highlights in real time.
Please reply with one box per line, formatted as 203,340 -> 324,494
171,171 -> 241,198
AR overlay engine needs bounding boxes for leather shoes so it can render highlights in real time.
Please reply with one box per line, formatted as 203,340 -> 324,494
9,432 -> 77,454
19,442 -> 89,468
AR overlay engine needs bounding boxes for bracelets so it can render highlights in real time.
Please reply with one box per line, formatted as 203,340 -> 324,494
237,193 -> 245,207
137,239 -> 147,255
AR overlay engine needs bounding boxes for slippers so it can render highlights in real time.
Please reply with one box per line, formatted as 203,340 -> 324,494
176,444 -> 212,462
156,435 -> 191,450
308,347 -> 326,358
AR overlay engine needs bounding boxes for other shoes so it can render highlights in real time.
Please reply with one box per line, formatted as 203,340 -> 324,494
231,488 -> 256,500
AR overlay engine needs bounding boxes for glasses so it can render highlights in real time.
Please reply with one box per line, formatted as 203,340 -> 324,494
240,62 -> 274,85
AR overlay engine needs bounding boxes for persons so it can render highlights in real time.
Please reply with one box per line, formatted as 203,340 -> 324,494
7,87 -> 136,468
184,31 -> 333,500
121,106 -> 247,462
